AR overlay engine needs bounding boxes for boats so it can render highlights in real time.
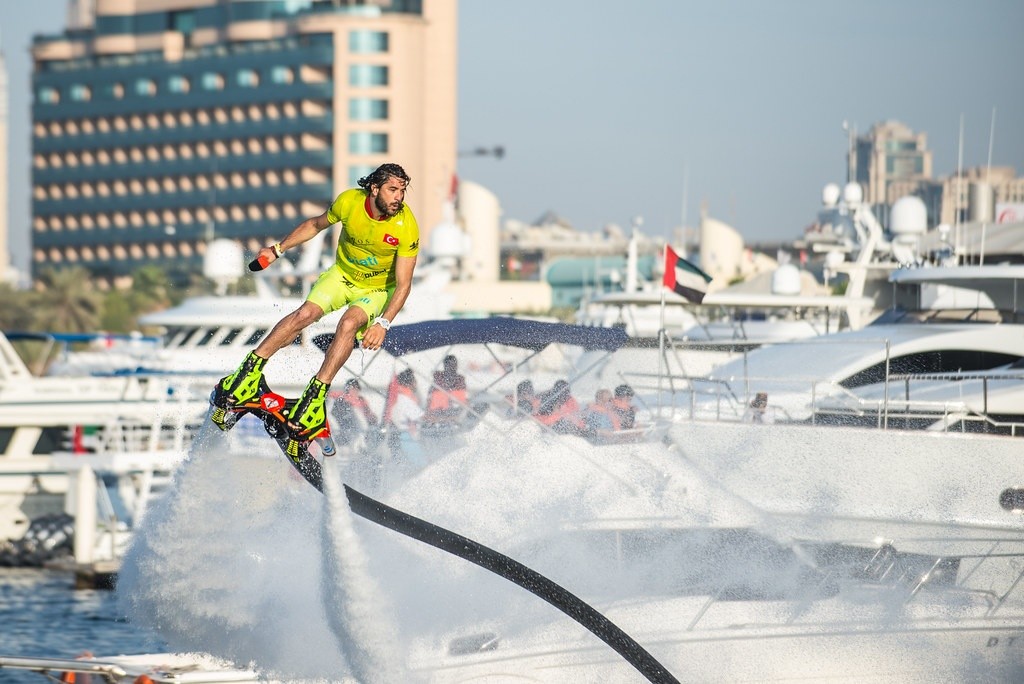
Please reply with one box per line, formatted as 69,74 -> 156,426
0,195 -> 1024,684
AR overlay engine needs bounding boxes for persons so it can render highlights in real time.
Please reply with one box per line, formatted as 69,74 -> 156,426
331,355 -> 638,462
208,163 -> 420,438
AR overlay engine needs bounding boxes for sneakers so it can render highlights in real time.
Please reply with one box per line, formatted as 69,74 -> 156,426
221,348 -> 269,405
287,375 -> 331,435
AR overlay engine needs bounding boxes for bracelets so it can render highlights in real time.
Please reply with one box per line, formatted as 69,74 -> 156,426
269,243 -> 285,259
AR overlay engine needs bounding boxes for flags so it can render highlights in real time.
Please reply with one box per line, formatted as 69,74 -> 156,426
663,244 -> 713,305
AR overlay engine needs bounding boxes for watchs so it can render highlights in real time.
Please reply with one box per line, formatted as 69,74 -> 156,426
372,317 -> 391,331
